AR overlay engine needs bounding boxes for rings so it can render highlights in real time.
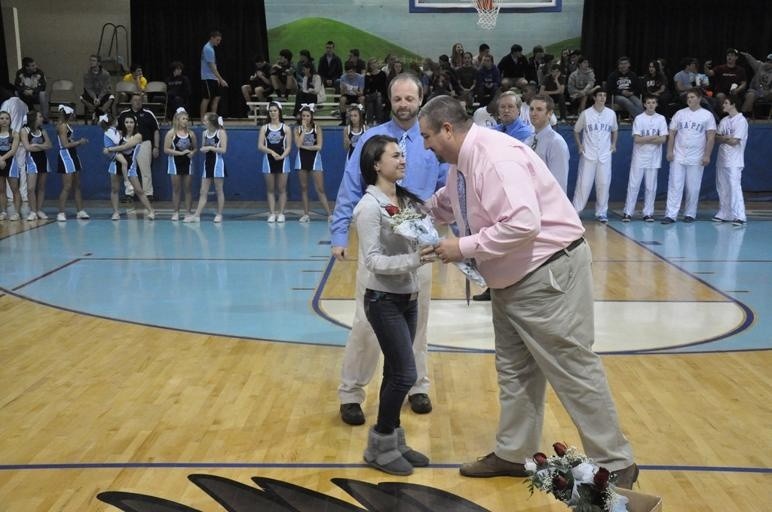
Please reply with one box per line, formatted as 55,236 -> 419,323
421,255 -> 424,261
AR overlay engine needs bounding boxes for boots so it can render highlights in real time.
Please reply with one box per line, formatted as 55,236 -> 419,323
363,425 -> 429,476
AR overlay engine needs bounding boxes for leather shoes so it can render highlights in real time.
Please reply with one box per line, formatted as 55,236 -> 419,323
474,287 -> 491,301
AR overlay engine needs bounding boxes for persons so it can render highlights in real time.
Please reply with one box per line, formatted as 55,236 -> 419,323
329,72 -> 461,425
352,134 -> 437,477
344,103 -> 366,153
470,84 -> 759,226
581,221 -> 750,340
257,100 -> 292,223
2,80 -> 90,226
98,92 -> 159,226
163,107 -> 197,222
418,94 -> 639,490
294,102 -> 332,222
2,31 -> 772,129
473,94 -> 571,302
182,112 -> 228,222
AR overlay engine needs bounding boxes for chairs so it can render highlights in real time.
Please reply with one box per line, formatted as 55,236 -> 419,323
48,80 -> 194,126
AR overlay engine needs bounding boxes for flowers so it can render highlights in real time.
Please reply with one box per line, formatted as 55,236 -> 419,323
523,442 -> 630,512
381,204 -> 486,288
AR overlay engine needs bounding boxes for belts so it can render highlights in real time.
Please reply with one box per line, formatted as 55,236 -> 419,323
366,288 -> 419,302
540,237 -> 584,267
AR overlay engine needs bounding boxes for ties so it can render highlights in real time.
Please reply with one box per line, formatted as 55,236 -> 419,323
532,135 -> 537,150
395,131 -> 408,185
456,170 -> 474,307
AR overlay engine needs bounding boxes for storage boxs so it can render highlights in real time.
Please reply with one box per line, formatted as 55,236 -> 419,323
614,487 -> 662,512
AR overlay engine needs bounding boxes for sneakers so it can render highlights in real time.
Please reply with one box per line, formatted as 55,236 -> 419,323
711,216 -> 746,225
267,213 -> 276,223
609,462 -> 639,489
277,213 -> 285,222
460,451 -> 534,477
77,210 -> 90,219
124,181 -> 134,191
214,214 -> 222,222
111,212 -> 120,220
0,210 -> 49,221
408,393 -> 432,414
298,214 -> 310,222
57,212 -> 67,221
328,215 -> 333,222
597,215 -> 696,224
147,211 -> 155,219
171,211 -> 200,222
341,403 -> 364,425
